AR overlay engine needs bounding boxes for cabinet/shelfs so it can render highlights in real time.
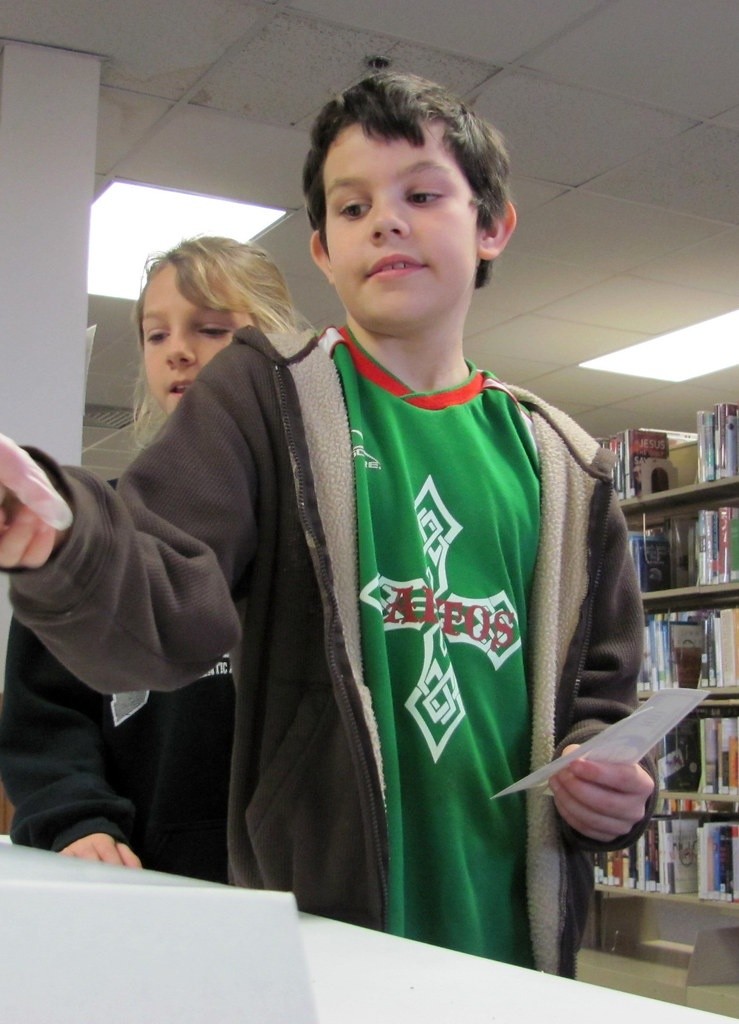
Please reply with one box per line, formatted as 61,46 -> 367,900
576,430 -> 739,1020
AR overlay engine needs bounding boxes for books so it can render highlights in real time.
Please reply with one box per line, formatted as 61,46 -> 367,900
595,814 -> 738,902
696,401 -> 739,483
637,607 -> 739,692
596,429 -> 671,500
657,708 -> 739,794
628,506 -> 739,593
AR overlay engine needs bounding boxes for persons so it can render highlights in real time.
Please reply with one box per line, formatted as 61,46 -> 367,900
0,71 -> 661,979
